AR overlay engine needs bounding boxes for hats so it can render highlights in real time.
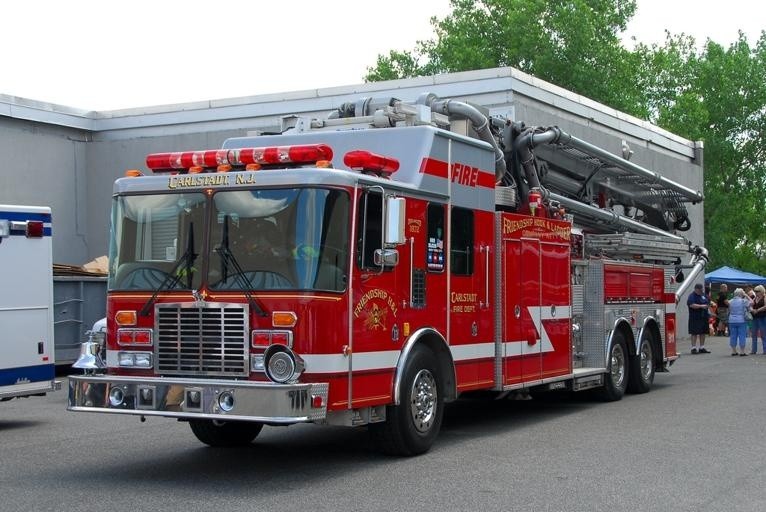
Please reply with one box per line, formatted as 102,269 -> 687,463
695,284 -> 703,289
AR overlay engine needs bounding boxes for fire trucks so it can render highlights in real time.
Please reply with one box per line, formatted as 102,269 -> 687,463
67,92 -> 709,457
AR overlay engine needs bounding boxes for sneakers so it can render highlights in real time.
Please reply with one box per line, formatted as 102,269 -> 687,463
699,348 -> 711,353
732,352 -> 747,356
691,348 -> 697,354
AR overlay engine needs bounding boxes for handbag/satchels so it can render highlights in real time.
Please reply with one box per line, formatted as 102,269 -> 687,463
745,311 -> 754,321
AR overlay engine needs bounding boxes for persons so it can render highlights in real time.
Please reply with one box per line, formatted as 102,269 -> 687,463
706,284 -> 766,356
687,284 -> 711,353
